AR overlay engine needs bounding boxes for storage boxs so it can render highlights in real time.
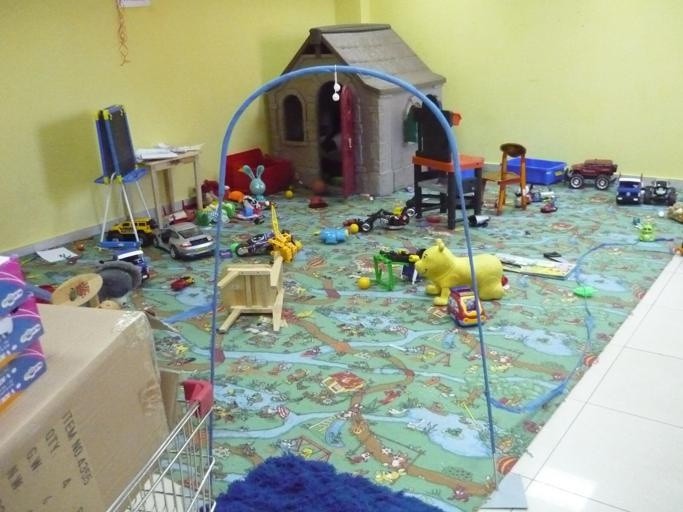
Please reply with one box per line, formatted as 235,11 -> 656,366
0,254 -> 181,511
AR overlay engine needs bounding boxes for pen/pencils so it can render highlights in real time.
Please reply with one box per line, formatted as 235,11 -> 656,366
500,261 -> 521,269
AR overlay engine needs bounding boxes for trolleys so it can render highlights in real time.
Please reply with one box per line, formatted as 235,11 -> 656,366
109,373 -> 219,512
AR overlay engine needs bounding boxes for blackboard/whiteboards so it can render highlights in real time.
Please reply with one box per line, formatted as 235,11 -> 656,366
103,103 -> 137,179
